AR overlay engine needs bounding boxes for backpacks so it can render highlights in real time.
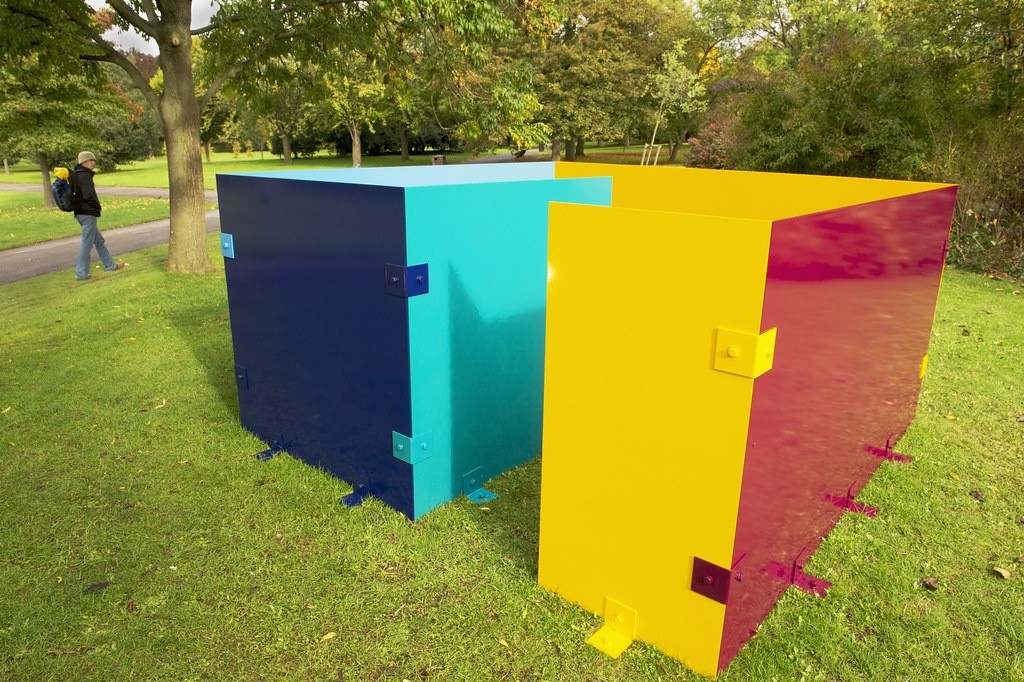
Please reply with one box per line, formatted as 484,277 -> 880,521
51,167 -> 87,212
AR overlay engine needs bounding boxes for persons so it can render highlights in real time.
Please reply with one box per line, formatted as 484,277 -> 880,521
72,151 -> 124,280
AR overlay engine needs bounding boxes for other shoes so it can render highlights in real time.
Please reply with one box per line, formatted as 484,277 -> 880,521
76,275 -> 95,281
113,263 -> 124,271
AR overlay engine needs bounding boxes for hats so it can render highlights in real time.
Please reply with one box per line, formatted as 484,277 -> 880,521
78,151 -> 96,164
